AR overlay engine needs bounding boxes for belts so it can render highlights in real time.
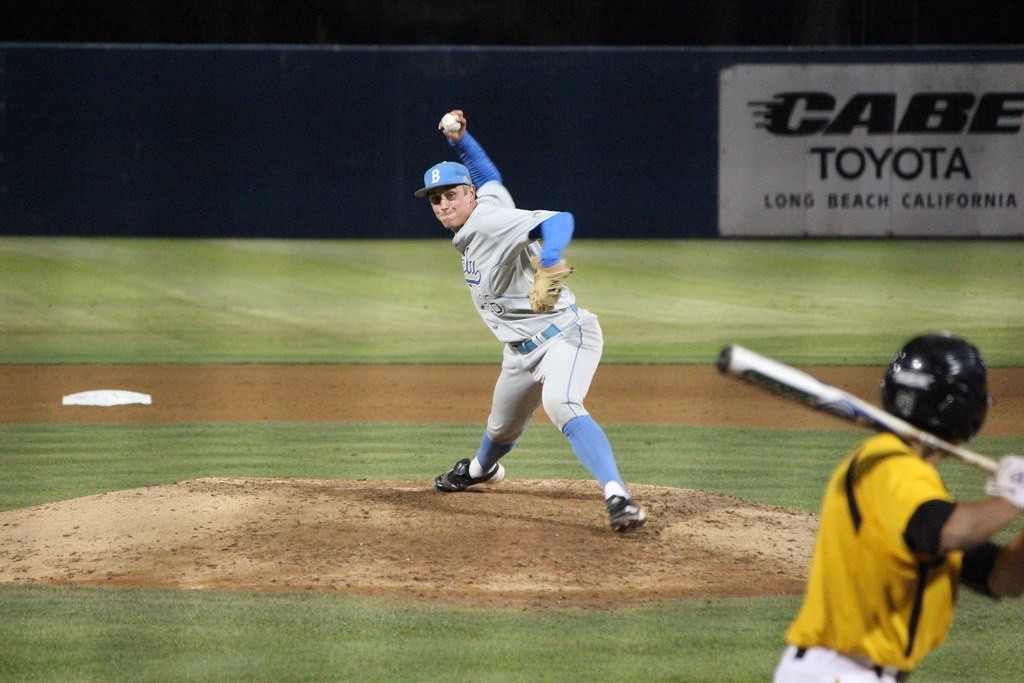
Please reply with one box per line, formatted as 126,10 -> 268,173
508,305 -> 579,353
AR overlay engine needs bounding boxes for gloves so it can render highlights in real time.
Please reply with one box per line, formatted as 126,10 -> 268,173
983,451 -> 1024,509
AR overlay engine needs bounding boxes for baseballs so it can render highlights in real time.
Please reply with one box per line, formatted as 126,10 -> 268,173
438,111 -> 463,132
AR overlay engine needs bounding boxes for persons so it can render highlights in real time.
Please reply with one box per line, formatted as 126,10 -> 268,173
411,110 -> 647,532
771,331 -> 1024,683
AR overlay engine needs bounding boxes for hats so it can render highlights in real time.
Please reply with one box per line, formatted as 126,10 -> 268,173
415,161 -> 472,199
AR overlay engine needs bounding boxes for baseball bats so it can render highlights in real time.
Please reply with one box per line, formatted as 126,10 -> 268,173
714,342 -> 1000,481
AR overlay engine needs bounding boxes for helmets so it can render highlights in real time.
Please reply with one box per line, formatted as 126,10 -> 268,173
881,331 -> 990,442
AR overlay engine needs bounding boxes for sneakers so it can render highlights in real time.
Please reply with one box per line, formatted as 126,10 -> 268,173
433,457 -> 506,493
605,495 -> 647,534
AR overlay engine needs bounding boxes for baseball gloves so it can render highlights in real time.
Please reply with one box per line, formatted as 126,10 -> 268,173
526,265 -> 568,316
